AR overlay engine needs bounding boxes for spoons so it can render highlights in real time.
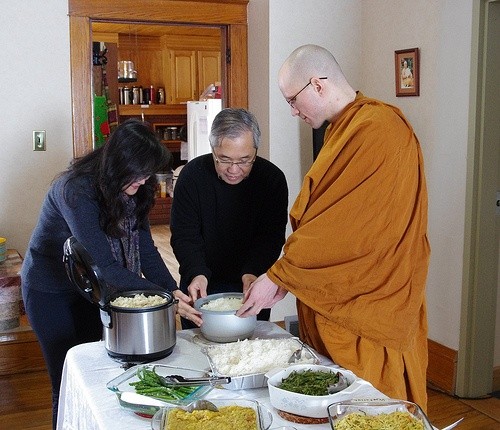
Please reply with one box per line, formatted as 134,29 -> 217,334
121,392 -> 220,413
287,338 -> 309,364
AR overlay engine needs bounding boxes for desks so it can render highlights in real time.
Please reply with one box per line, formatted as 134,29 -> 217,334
0,249 -> 23,332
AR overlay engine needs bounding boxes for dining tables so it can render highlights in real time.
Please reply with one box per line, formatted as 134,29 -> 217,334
56,320 -> 439,430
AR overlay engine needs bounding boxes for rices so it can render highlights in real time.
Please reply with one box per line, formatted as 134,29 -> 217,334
208,337 -> 314,377
200,297 -> 244,311
110,293 -> 167,308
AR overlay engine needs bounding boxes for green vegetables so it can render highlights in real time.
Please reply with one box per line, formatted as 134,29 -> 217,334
275,368 -> 350,396
128,366 -> 198,403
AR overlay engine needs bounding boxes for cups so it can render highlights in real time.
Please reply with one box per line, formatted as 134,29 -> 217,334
121,87 -> 131,105
144,88 -> 154,104
132,87 -> 143,104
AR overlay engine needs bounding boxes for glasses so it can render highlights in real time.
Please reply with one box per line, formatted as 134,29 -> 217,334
287,76 -> 328,108
212,147 -> 258,166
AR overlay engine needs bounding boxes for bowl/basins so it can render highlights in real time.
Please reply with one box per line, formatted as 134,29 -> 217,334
326,399 -> 434,430
151,397 -> 273,430
193,291 -> 257,343
264,366 -> 363,418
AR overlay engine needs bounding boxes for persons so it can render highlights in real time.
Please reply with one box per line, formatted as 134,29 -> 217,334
401,60 -> 413,79
18,119 -> 202,430
233,42 -> 430,423
169,106 -> 290,330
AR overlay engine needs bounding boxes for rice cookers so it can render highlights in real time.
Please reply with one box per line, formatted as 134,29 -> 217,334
61,236 -> 178,369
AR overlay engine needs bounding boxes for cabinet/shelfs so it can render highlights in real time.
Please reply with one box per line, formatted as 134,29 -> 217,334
93,23 -> 223,222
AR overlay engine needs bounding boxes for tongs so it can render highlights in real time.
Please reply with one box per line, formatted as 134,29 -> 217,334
162,374 -> 231,388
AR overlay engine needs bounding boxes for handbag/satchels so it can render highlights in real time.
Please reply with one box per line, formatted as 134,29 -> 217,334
63,236 -> 99,303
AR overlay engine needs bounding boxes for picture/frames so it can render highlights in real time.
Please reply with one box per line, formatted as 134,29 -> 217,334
394,48 -> 420,97
